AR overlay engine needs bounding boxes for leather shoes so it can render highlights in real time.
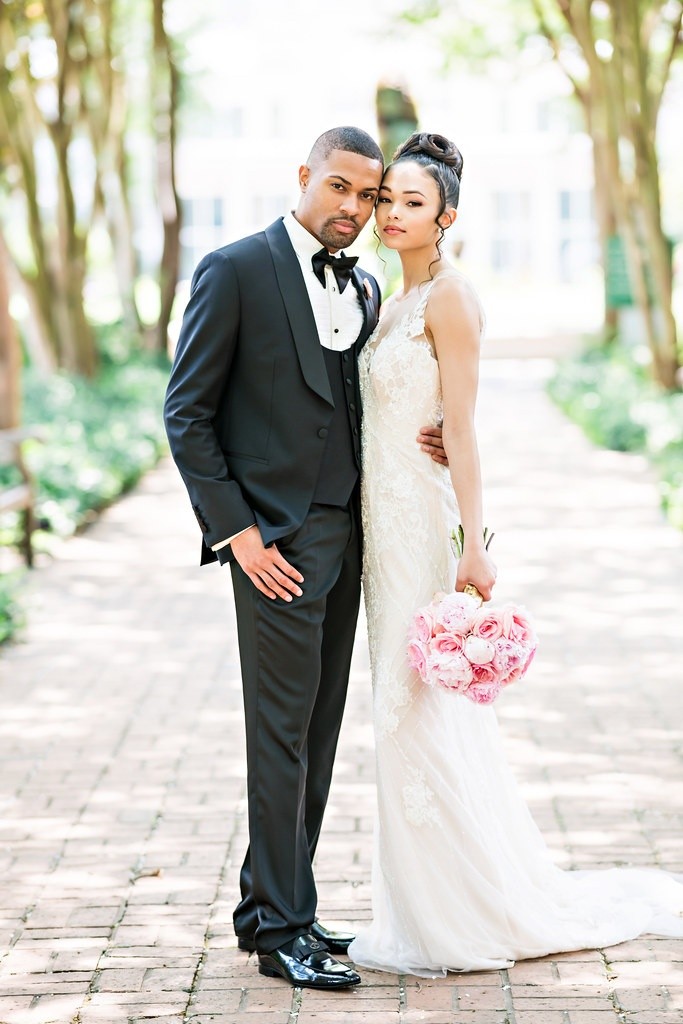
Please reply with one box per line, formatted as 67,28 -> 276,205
257,933 -> 361,989
238,920 -> 356,955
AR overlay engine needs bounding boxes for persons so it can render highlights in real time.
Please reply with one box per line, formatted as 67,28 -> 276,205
348,136 -> 683,978
163,128 -> 447,987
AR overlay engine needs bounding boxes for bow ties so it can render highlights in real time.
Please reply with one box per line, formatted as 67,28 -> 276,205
311,247 -> 359,295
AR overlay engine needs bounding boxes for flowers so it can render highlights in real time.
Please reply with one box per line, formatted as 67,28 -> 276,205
407,526 -> 540,706
